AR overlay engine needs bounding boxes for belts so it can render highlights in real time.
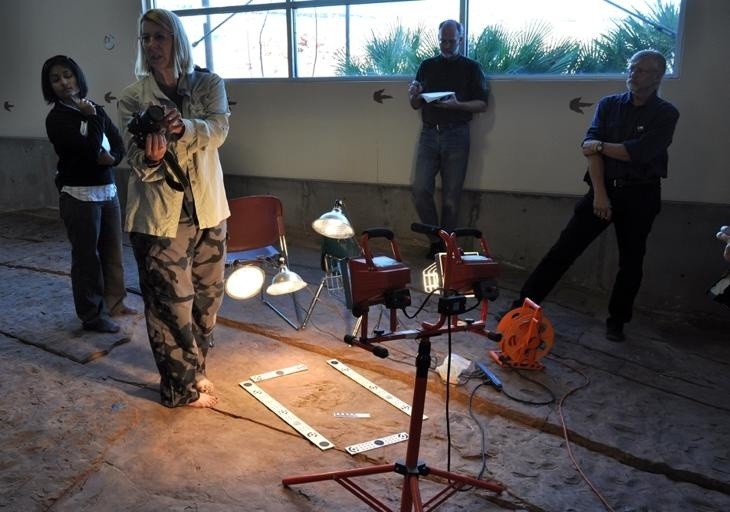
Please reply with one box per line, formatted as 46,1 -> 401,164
423,122 -> 460,130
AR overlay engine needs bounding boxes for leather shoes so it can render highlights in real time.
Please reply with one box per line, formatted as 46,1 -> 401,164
121,305 -> 138,315
101,320 -> 120,332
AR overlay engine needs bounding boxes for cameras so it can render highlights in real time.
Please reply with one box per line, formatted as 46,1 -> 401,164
128,106 -> 164,149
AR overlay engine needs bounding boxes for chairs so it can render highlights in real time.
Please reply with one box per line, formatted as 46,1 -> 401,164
227,195 -> 304,333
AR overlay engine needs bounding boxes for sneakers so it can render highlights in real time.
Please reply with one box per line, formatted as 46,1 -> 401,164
498,299 -> 522,317
605,316 -> 625,342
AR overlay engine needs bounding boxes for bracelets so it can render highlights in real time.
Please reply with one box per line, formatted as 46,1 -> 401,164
595,140 -> 603,150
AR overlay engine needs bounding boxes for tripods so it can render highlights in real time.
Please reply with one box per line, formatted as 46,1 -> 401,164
281,346 -> 505,511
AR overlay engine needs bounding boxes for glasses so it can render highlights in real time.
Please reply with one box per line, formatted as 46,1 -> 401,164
137,31 -> 173,44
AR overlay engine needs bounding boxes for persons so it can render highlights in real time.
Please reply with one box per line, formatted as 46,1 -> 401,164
113,7 -> 228,408
40,53 -> 137,334
494,48 -> 683,343
406,19 -> 490,257
